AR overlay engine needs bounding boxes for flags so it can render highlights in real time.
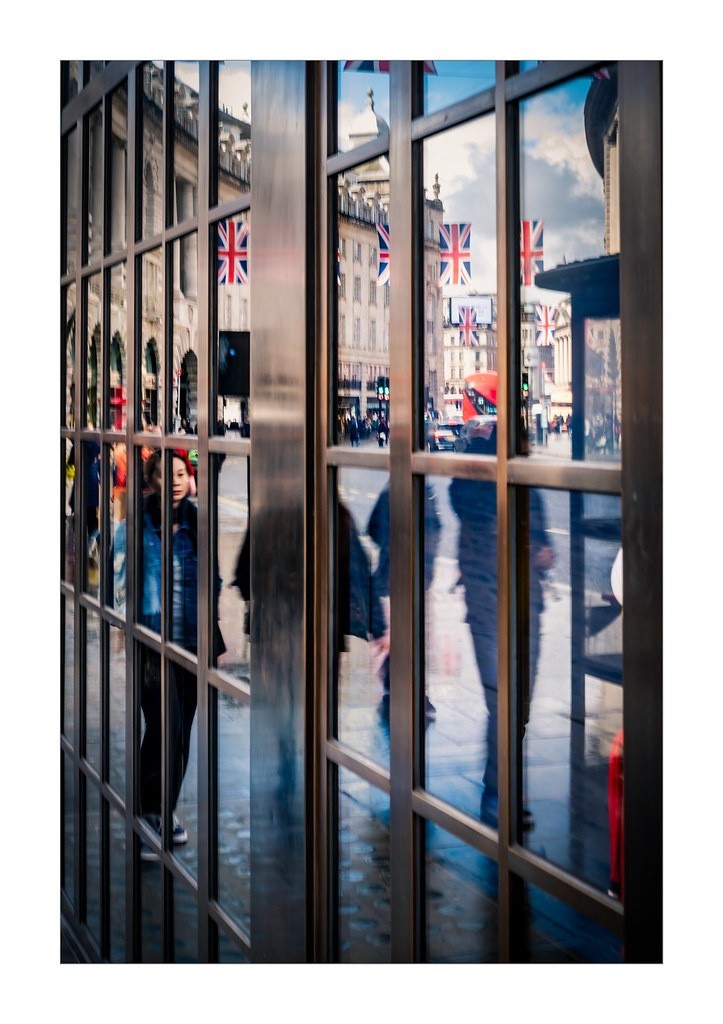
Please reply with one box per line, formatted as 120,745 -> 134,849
459,306 -> 479,347
520,221 -> 544,286
344,61 -> 438,76
337,249 -> 342,286
538,60 -> 609,80
440,224 -> 471,287
215,220 -> 249,286
535,305 -> 556,346
376,224 -> 390,287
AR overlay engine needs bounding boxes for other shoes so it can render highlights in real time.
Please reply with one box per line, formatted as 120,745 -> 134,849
476,792 -> 535,834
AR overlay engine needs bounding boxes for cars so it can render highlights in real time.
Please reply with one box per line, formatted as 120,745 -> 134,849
425,419 -> 465,454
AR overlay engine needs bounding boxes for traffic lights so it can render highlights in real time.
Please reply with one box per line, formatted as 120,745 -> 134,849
520,371 -> 531,403
217,329 -> 249,398
377,375 -> 390,403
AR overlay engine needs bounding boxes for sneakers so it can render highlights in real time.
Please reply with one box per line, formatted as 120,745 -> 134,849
138,809 -> 188,862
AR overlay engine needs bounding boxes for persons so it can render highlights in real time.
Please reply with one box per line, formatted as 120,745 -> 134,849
232,504 -> 387,653
604,413 -> 619,453
68,428 -> 100,569
343,416 -> 390,448
177,416 -> 253,498
550,413 -> 572,435
368,472 -> 443,713
112,449 -> 220,861
450,415 -> 557,830
